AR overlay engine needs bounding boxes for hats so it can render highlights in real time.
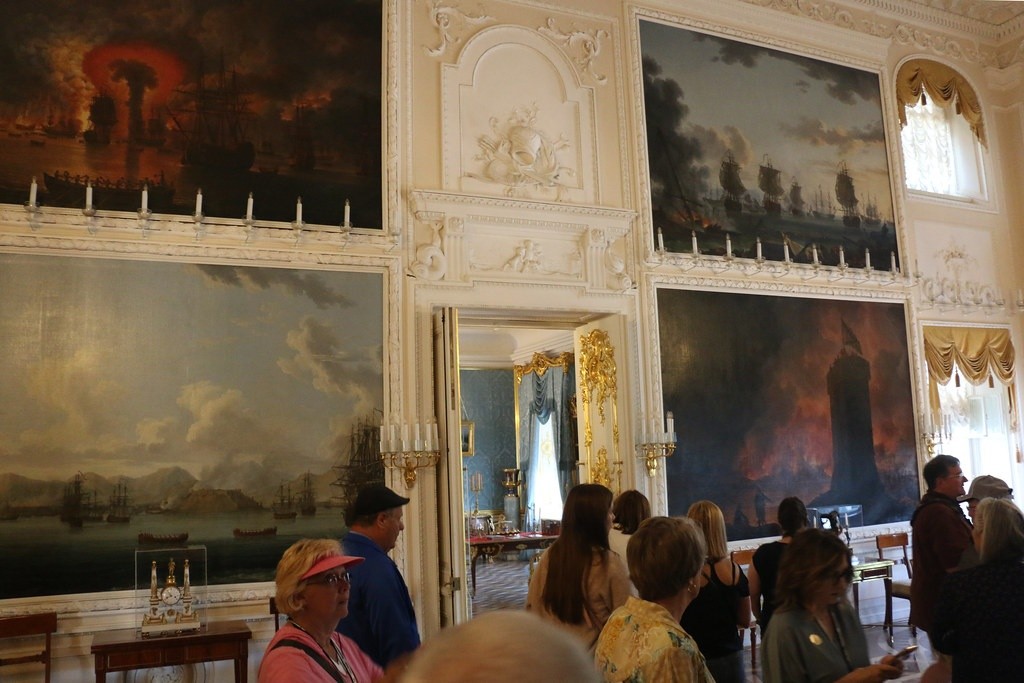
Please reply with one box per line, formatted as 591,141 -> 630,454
356,487 -> 410,514
956,474 -> 1013,503
300,551 -> 363,583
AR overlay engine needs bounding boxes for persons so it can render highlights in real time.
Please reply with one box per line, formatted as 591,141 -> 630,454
592,515 -> 718,683
394,609 -> 602,683
925,497 -> 1023,683
760,527 -> 905,683
678,500 -> 753,683
951,474 -> 1016,527
748,496 -> 822,642
908,454 -> 976,682
251,536 -> 386,683
522,483 -> 631,662
338,483 -> 423,671
606,489 -> 656,598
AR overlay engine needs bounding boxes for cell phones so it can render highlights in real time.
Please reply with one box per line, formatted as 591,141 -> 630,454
885,645 -> 918,666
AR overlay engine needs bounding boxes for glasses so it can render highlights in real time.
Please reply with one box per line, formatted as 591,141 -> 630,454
304,570 -> 350,586
966,506 -> 976,511
944,472 -> 964,481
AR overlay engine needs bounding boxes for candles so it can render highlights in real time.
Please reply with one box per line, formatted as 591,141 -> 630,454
379,415 -> 440,451
635,411 -> 675,443
471,472 -> 483,490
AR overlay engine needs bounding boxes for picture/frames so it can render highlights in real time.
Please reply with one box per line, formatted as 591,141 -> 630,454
636,13 -> 905,277
0,0 -> 390,237
0,245 -> 389,619
461,420 -> 475,457
653,282 -> 927,549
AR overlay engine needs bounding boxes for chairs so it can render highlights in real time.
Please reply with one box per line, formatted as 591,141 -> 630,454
876,532 -> 918,638
465,514 -> 495,565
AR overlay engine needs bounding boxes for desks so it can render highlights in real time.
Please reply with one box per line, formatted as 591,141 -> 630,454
740,557 -> 895,675
0,610 -> 58,683
91,619 -> 252,683
465,531 -> 561,599
